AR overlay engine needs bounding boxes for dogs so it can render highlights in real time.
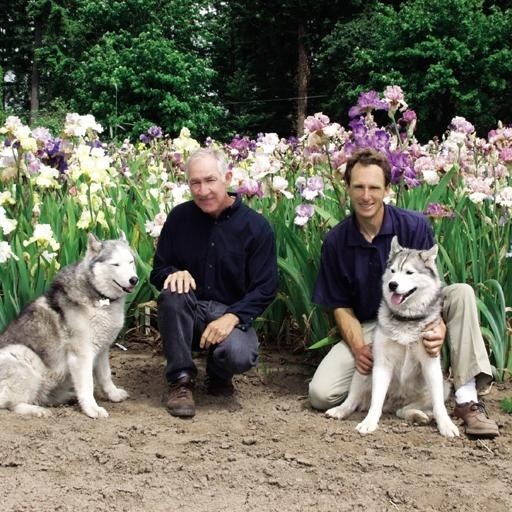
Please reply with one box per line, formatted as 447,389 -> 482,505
0,228 -> 140,420
324,232 -> 461,442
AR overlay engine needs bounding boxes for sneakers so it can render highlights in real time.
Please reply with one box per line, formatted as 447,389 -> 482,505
207,359 -> 234,396
167,378 -> 196,417
455,400 -> 500,436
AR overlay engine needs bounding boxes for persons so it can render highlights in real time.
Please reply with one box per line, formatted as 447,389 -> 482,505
147,147 -> 279,419
306,148 -> 503,440
